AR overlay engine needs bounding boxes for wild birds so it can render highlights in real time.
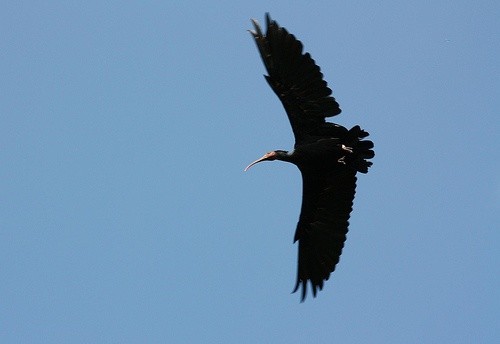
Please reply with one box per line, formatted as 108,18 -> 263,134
242,11 -> 376,304
